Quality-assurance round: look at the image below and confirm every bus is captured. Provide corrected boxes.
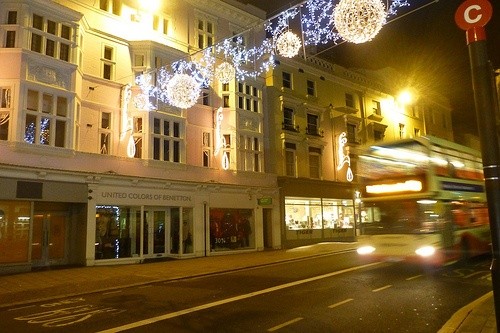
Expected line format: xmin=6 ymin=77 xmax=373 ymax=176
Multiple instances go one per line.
xmin=353 ymin=132 xmax=493 ymax=272
